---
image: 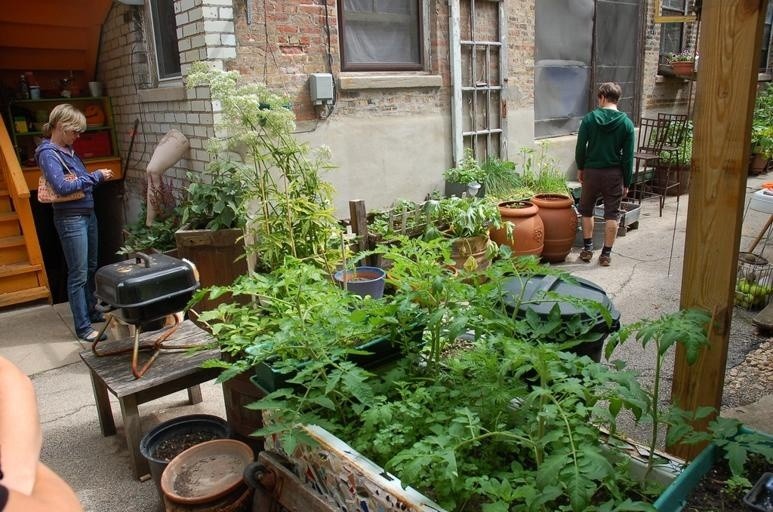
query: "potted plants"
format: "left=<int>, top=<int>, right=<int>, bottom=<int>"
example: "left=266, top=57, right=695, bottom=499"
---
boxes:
left=651, top=401, right=773, bottom=512
left=241, top=242, right=692, bottom=512
left=651, top=79, right=773, bottom=195
left=175, top=158, right=251, bottom=325
left=115, top=171, right=178, bottom=259
left=742, top=472, right=773, bottom=512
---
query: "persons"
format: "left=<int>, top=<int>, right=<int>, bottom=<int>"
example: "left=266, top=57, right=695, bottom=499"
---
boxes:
left=575, top=81, right=635, bottom=266
left=34, top=104, right=113, bottom=341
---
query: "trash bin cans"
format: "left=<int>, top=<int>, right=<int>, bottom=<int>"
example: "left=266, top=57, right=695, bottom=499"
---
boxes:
left=492, top=270, right=620, bottom=424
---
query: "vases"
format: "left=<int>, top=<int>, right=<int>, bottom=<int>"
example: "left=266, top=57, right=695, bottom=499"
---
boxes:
left=87, top=81, right=103, bottom=96
left=671, top=62, right=694, bottom=76
left=138, top=413, right=255, bottom=512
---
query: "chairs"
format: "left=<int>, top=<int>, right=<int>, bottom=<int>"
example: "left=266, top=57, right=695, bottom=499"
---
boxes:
left=623, top=117, right=671, bottom=217
left=639, top=113, right=688, bottom=208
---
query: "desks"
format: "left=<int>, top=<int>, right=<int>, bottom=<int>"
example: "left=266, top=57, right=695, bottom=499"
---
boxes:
left=79, top=323, right=222, bottom=480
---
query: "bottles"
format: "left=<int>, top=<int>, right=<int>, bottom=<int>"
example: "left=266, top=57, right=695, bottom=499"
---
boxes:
left=18, top=74, right=30, bottom=100
left=23, top=71, right=41, bottom=100
left=57, top=78, right=72, bottom=98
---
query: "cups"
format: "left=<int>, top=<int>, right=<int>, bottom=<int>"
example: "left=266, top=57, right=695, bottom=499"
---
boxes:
left=86, top=82, right=104, bottom=98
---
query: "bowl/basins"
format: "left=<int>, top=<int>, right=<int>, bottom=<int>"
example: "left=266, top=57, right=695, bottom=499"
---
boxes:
left=31, top=122, right=47, bottom=131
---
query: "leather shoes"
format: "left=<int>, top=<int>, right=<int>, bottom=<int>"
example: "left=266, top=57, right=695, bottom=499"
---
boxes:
left=78, top=316, right=108, bottom=342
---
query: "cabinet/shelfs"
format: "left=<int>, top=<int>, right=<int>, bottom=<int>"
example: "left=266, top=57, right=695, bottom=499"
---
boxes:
left=5, top=96, right=123, bottom=193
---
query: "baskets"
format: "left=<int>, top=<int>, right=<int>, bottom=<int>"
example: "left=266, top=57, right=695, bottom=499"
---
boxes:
left=732, top=255, right=773, bottom=312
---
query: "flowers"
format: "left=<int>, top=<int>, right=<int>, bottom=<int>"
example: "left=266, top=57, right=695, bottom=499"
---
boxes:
left=663, top=48, right=694, bottom=61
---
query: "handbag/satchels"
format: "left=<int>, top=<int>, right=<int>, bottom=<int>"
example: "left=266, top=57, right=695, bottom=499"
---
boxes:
left=36, top=174, right=85, bottom=204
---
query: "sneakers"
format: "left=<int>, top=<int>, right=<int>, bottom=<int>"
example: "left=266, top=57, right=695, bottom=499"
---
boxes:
left=598, top=254, right=611, bottom=266
left=580, top=249, right=592, bottom=261
